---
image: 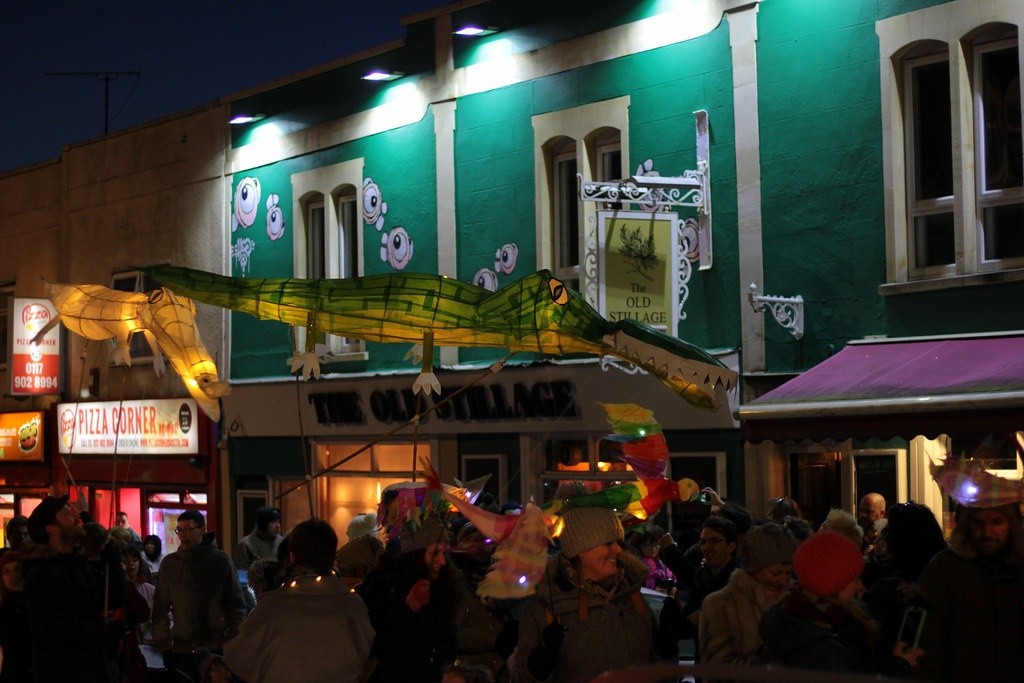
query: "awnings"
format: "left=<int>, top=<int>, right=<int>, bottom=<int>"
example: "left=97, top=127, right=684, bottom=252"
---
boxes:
left=733, top=329, right=1024, bottom=421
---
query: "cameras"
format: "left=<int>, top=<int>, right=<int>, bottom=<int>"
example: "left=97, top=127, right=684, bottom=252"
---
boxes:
left=700, top=489, right=706, bottom=503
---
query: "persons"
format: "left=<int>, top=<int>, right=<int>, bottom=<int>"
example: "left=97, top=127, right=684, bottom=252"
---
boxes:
left=0, top=472, right=1024, bottom=683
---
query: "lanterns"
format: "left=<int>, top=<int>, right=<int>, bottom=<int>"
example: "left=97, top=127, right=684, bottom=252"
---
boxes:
left=30, top=262, right=738, bottom=422
left=371, top=403, right=699, bottom=604
left=929, top=431, right=1024, bottom=509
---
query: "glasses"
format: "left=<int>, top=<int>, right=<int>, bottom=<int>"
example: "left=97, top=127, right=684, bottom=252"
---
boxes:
left=697, top=537, right=725, bottom=545
left=175, top=527, right=197, bottom=534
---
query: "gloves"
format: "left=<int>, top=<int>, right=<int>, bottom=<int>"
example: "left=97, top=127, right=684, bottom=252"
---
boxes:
left=542, top=619, right=570, bottom=655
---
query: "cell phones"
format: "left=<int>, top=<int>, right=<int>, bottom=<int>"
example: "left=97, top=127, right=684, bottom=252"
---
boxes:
left=896, top=605, right=926, bottom=653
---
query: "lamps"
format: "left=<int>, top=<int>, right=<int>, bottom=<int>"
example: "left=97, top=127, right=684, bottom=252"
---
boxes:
left=228, top=113, right=265, bottom=126
left=450, top=22, right=499, bottom=37
left=360, top=68, right=403, bottom=82
left=286, top=313, right=320, bottom=382
left=413, top=330, right=441, bottom=397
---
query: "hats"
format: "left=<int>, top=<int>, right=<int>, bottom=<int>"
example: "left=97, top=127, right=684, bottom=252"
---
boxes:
left=192, top=646, right=224, bottom=683
left=817, top=507, right=863, bottom=547
left=450, top=516, right=468, bottom=536
left=477, top=492, right=501, bottom=515
left=401, top=505, right=448, bottom=552
left=744, top=523, right=798, bottom=576
left=248, top=555, right=286, bottom=590
left=247, top=507, right=281, bottom=523
left=27, top=495, right=69, bottom=544
left=552, top=480, right=625, bottom=559
left=0, top=551, right=27, bottom=575
left=345, top=513, right=376, bottom=537
left=792, top=531, right=864, bottom=597
left=340, top=534, right=373, bottom=566
